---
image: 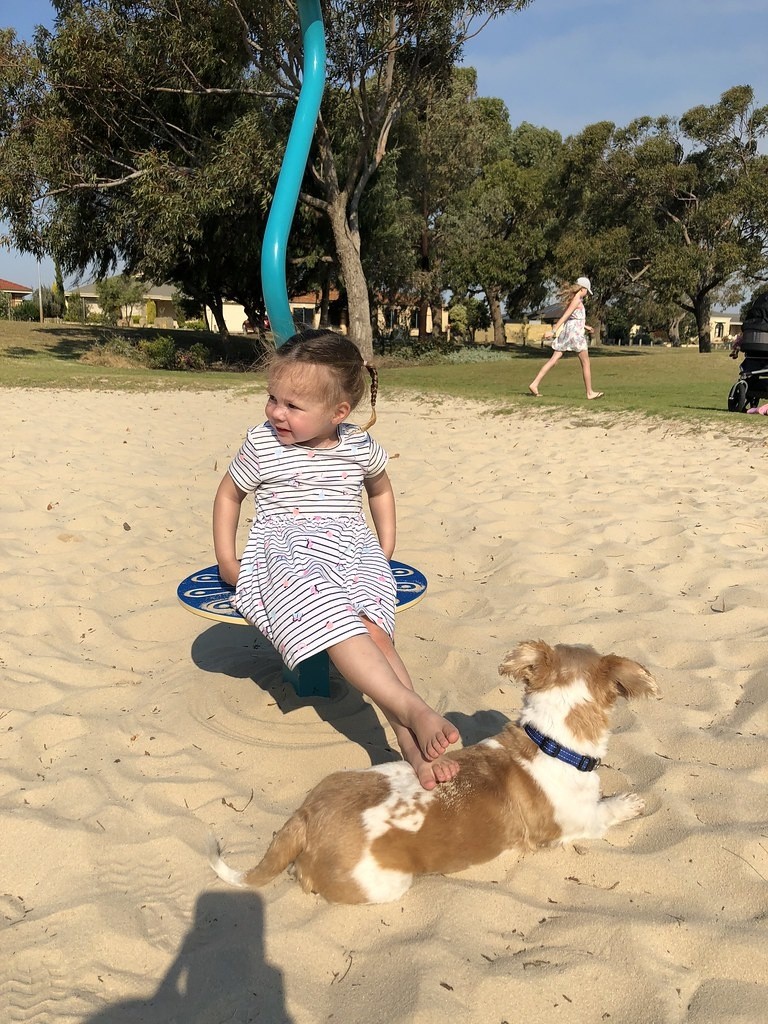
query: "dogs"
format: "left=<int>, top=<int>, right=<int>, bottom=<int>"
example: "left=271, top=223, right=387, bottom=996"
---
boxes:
left=206, top=639, right=659, bottom=907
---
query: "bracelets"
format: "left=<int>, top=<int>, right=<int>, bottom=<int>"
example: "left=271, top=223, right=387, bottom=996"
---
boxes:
left=551, top=330, right=556, bottom=333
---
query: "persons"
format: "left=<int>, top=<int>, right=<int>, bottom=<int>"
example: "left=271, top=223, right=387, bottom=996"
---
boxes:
left=528, top=276, right=605, bottom=400
left=211, top=327, right=461, bottom=792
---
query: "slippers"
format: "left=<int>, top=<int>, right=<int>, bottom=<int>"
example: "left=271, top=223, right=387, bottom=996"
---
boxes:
left=528, top=393, right=540, bottom=397
left=589, top=392, right=604, bottom=400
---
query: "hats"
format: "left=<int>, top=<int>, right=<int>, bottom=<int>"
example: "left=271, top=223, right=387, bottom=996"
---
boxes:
left=575, top=277, right=593, bottom=295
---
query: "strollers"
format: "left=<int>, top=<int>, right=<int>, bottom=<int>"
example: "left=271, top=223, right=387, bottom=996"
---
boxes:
left=727, top=290, right=768, bottom=412
